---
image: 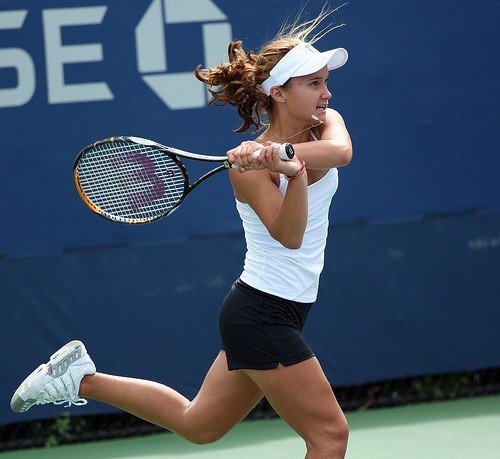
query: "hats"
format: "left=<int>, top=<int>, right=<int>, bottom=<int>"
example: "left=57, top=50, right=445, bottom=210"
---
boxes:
left=260, top=43, right=349, bottom=96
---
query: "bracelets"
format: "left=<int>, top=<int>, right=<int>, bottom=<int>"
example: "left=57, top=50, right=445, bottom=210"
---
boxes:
left=284, top=159, right=306, bottom=181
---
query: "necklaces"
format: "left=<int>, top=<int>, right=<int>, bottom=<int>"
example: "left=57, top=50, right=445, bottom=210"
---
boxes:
left=270, top=114, right=323, bottom=139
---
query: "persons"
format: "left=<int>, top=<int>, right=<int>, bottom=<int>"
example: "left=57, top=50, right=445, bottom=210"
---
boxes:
left=10, top=37, right=354, bottom=459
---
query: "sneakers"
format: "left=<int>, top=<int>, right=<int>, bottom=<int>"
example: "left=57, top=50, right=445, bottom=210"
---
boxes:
left=10, top=341, right=96, bottom=414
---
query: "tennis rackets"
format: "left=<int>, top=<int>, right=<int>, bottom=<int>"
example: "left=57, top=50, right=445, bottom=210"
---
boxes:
left=70, top=134, right=296, bottom=227
